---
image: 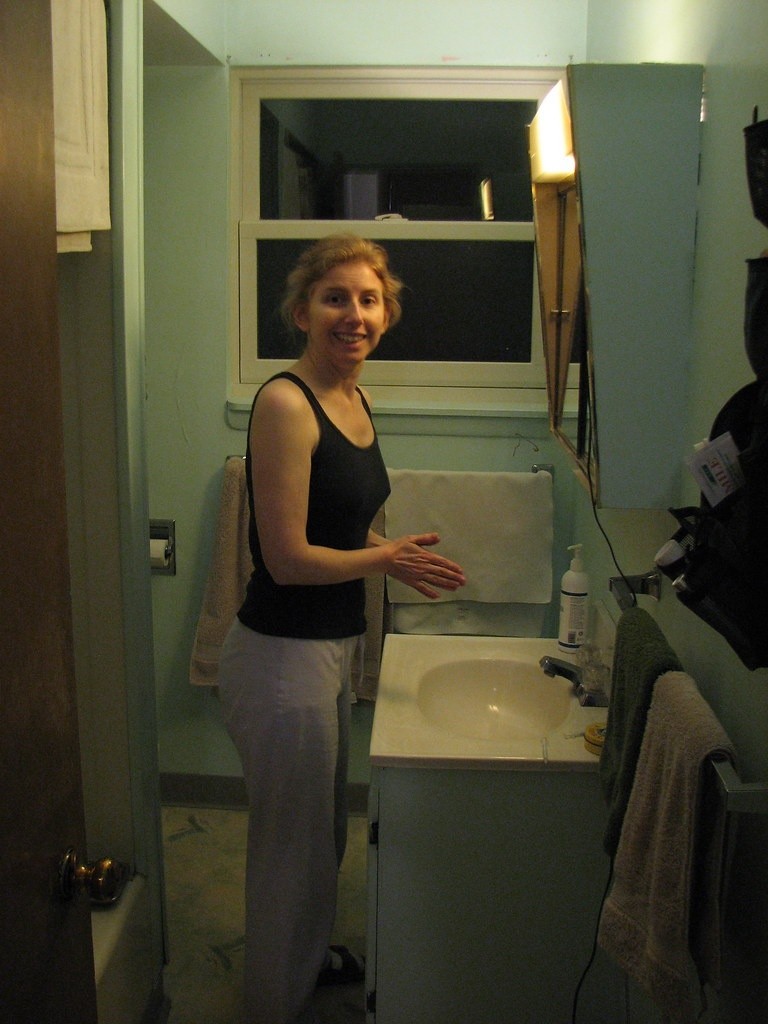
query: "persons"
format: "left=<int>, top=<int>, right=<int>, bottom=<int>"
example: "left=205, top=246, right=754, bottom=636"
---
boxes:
left=216, top=238, right=468, bottom=1024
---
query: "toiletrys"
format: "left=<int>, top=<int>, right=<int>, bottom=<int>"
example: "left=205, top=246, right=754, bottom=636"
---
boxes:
left=557, top=542, right=592, bottom=653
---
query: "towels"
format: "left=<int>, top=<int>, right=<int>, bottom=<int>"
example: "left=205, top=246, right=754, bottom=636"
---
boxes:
left=190, top=455, right=557, bottom=705
left=575, top=606, right=743, bottom=1024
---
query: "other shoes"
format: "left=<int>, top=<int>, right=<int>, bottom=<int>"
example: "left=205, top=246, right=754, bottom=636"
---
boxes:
left=316, top=944, right=364, bottom=986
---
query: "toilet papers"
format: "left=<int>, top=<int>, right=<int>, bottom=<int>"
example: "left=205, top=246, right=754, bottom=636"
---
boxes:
left=150, top=536, right=170, bottom=565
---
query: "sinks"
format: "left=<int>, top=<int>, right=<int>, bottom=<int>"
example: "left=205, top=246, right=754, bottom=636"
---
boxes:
left=416, top=659, right=573, bottom=742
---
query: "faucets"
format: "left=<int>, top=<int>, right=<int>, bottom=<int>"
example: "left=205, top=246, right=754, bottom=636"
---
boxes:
left=539, top=655, right=586, bottom=686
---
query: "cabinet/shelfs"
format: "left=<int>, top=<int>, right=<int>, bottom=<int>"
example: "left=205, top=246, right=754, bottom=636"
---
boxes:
left=0, top=0, right=169, bottom=1024
left=524, top=62, right=706, bottom=513
